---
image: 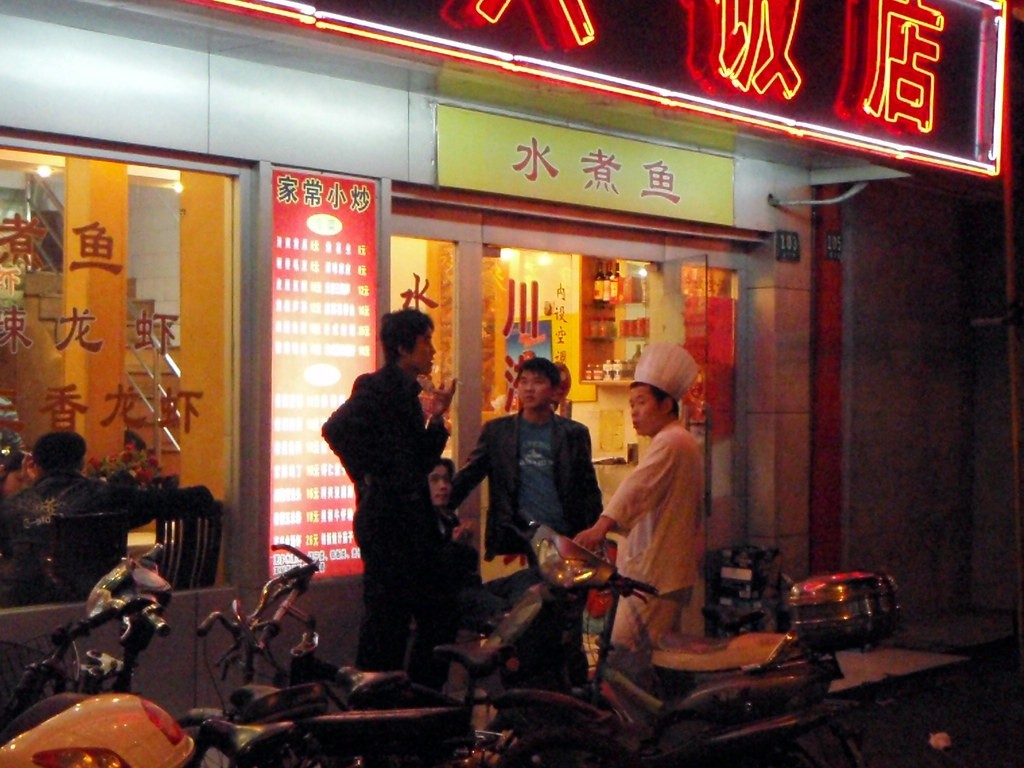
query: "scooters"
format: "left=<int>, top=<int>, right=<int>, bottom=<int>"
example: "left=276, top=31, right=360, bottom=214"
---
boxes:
left=430, top=517, right=903, bottom=767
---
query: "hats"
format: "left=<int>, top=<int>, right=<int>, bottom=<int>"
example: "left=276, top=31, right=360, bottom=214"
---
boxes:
left=632, top=343, right=698, bottom=402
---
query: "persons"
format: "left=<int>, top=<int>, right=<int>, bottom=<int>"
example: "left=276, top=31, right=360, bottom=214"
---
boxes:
left=321, top=309, right=480, bottom=691
left=444, top=357, right=604, bottom=569
left=573, top=341, right=707, bottom=646
left=0, top=429, right=153, bottom=610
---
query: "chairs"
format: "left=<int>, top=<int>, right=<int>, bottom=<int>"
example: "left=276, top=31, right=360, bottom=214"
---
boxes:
left=152, top=486, right=225, bottom=589
left=50, top=510, right=130, bottom=604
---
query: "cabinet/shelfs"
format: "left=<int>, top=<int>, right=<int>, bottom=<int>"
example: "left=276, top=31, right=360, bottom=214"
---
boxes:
left=576, top=255, right=652, bottom=387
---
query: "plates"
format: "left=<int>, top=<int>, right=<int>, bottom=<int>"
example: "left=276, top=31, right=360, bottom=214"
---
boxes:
left=307, top=214, right=342, bottom=235
left=303, top=363, right=341, bottom=388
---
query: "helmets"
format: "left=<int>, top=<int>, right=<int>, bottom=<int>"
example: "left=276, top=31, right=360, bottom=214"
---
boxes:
left=0, top=428, right=24, bottom=480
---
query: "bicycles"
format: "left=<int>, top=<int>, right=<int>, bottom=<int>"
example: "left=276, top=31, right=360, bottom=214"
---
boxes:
left=0, top=537, right=799, bottom=768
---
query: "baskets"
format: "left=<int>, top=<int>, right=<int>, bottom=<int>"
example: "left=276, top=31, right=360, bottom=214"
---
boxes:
left=0, top=640, right=51, bottom=722
